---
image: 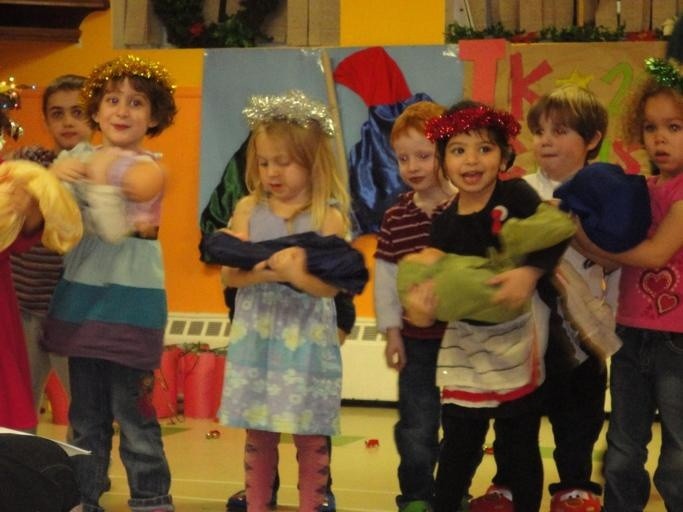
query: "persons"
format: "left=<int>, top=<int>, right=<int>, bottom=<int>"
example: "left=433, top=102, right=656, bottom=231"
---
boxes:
left=547, top=53, right=683, bottom=511
left=514, top=75, right=623, bottom=512
left=393, top=98, right=573, bottom=510
left=0, top=76, right=47, bottom=435
left=5, top=73, right=93, bottom=428
left=372, top=96, right=478, bottom=512
left=39, top=52, right=177, bottom=510
left=197, top=85, right=370, bottom=512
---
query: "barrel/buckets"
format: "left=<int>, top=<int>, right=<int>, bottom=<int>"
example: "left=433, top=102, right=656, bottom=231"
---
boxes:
left=183, top=349, right=227, bottom=422
left=146, top=345, right=183, bottom=421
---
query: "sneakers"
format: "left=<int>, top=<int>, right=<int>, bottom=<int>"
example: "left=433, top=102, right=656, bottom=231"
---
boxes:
left=470, top=483, right=515, bottom=512
left=549, top=482, right=599, bottom=511
left=318, top=492, right=335, bottom=510
left=226, top=489, right=277, bottom=509
